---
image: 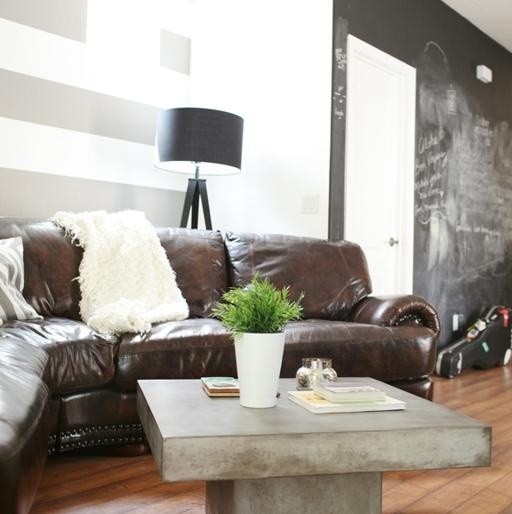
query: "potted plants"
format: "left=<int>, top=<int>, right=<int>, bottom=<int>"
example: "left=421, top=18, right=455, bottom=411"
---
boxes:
left=208, top=266, right=306, bottom=407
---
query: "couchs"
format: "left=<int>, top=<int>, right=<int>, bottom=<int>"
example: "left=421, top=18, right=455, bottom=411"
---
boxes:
left=0, top=220, right=442, bottom=513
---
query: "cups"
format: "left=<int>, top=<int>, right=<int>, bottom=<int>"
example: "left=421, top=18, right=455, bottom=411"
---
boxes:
left=301, top=356, right=332, bottom=385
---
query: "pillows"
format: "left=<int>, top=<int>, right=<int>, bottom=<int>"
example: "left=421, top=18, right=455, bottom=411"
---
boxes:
left=0, top=235, right=45, bottom=320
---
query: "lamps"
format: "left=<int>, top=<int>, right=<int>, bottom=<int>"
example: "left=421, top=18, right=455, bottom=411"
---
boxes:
left=152, top=105, right=246, bottom=229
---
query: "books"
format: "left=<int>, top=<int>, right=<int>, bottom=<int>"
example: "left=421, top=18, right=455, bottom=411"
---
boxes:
left=314, top=386, right=386, bottom=401
left=290, top=390, right=407, bottom=415
left=200, top=376, right=239, bottom=397
